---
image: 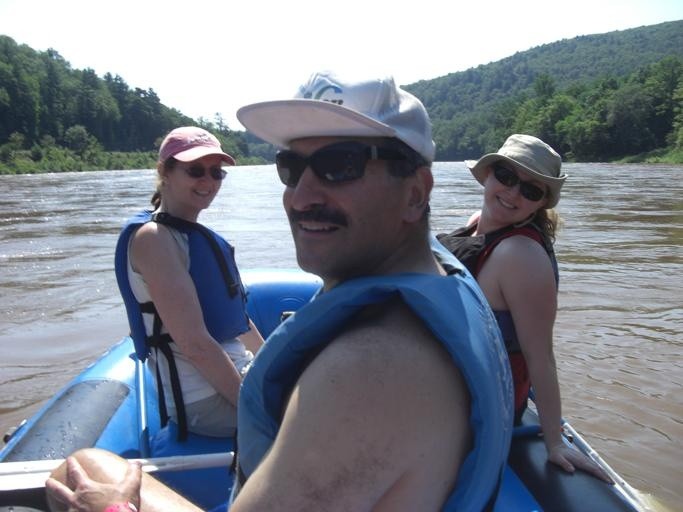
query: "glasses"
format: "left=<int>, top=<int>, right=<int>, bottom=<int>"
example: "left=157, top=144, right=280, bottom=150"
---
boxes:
left=275, top=140, right=408, bottom=189
left=185, top=165, right=228, bottom=180
left=493, top=164, right=544, bottom=201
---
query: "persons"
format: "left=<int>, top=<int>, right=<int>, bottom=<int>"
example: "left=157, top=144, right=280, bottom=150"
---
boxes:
left=45, top=70, right=516, bottom=512
left=114, top=127, right=265, bottom=440
left=433, top=134, right=617, bottom=484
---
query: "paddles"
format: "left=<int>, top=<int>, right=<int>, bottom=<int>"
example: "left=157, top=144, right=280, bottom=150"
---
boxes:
left=1, top=452, right=233, bottom=491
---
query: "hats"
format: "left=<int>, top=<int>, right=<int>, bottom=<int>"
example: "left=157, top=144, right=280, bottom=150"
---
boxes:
left=236, top=60, right=437, bottom=162
left=464, top=134, right=568, bottom=209
left=159, top=125, right=236, bottom=165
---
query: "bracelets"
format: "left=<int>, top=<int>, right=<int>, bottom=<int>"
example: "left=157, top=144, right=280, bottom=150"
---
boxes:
left=105, top=501, right=138, bottom=512
